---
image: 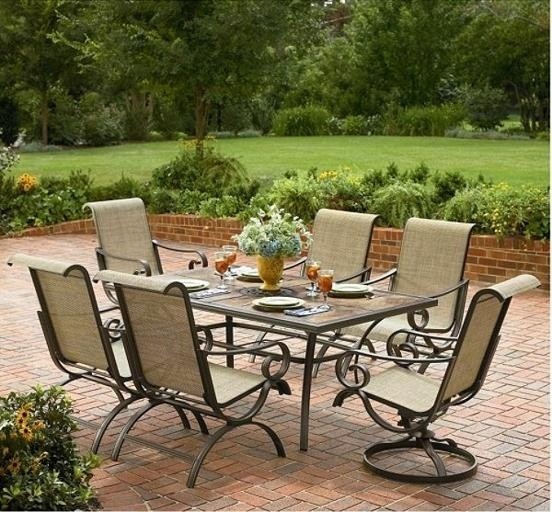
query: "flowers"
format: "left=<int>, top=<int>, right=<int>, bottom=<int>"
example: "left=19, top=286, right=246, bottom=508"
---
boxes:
left=232, top=202, right=312, bottom=256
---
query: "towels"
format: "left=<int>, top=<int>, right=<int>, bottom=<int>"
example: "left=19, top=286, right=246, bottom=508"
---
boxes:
left=283, top=303, right=331, bottom=318
left=188, top=282, right=230, bottom=298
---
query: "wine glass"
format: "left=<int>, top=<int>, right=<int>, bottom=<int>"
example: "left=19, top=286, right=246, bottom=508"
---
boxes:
left=214, top=252, right=229, bottom=289
left=304, top=261, right=321, bottom=297
left=223, top=245, right=237, bottom=281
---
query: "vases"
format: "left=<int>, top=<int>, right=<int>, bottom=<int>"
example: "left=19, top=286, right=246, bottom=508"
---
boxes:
left=255, top=250, right=287, bottom=293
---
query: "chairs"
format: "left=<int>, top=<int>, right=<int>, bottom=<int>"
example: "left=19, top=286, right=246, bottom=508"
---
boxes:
left=334, top=215, right=482, bottom=388
left=6, top=251, right=215, bottom=460
left=330, top=271, right=544, bottom=489
left=246, top=207, right=379, bottom=380
left=92, top=266, right=295, bottom=492
left=79, top=194, right=210, bottom=343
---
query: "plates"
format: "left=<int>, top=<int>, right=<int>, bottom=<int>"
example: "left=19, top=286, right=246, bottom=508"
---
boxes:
left=170, top=268, right=373, bottom=309
